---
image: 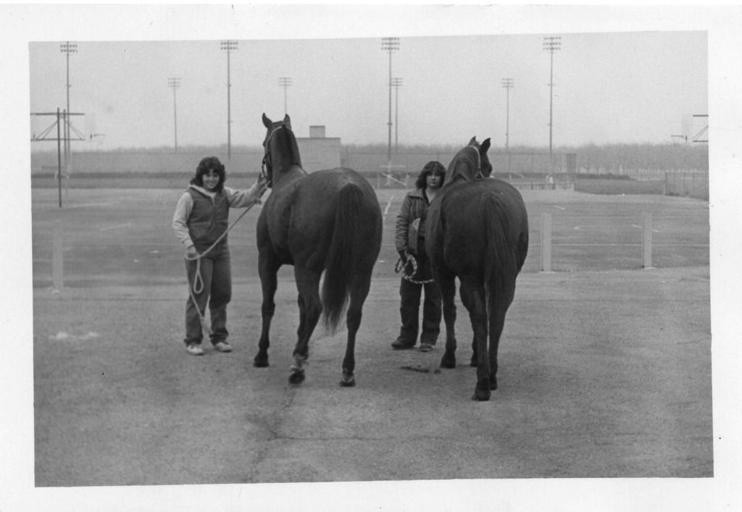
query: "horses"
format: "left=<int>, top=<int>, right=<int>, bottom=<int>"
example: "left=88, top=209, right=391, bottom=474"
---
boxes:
left=421, top=133, right=531, bottom=403
left=251, top=111, right=385, bottom=387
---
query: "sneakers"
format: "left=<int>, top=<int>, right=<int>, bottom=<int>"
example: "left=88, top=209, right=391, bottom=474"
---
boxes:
left=214, top=342, right=233, bottom=351
left=391, top=337, right=415, bottom=350
left=420, top=342, right=435, bottom=352
left=186, top=343, right=204, bottom=356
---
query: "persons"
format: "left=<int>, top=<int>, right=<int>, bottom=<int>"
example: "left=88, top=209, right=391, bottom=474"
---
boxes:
left=390, top=161, right=448, bottom=352
left=172, top=156, right=271, bottom=356
